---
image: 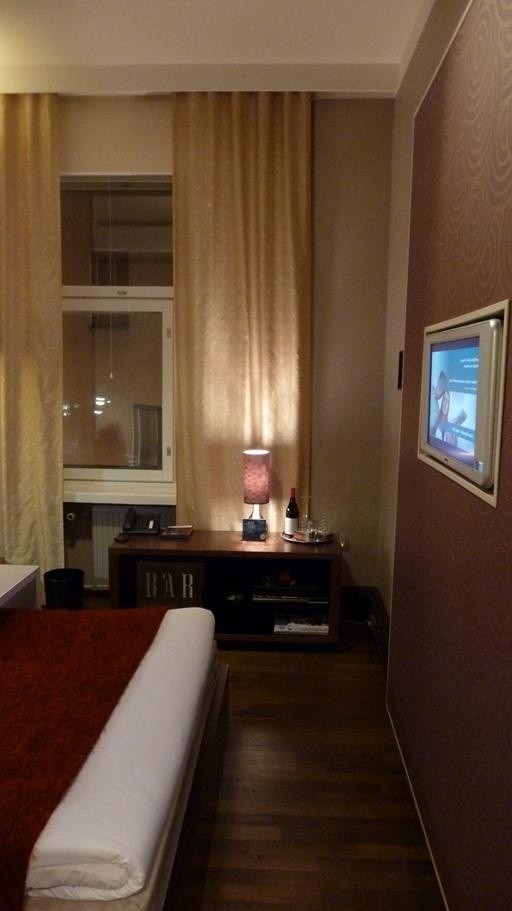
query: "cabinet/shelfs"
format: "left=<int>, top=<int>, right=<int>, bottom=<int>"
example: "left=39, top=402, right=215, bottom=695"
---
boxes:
left=106, top=528, right=344, bottom=653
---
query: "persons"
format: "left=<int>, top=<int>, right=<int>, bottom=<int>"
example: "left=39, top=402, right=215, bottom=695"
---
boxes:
left=429, top=370, right=467, bottom=445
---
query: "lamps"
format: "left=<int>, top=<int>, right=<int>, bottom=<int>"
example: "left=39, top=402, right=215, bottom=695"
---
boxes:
left=239, top=449, right=271, bottom=542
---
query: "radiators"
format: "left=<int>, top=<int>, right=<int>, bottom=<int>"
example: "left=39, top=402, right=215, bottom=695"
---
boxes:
left=91, top=506, right=174, bottom=579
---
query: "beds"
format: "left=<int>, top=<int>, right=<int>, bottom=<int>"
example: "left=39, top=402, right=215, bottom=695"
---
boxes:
left=1, top=606, right=230, bottom=911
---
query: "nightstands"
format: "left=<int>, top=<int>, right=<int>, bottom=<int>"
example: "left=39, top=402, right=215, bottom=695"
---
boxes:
left=0, top=563, right=42, bottom=608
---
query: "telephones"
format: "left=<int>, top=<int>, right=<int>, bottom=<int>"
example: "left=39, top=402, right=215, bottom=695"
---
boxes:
left=123, top=508, right=161, bottom=534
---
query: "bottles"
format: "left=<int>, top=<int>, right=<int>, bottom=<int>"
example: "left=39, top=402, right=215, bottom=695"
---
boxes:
left=284, top=487, right=299, bottom=539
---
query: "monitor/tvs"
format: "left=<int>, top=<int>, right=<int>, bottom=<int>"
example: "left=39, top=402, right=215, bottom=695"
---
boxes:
left=420, top=317, right=503, bottom=491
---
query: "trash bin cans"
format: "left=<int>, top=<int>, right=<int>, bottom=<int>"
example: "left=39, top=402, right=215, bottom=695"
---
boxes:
left=44, top=568, right=85, bottom=611
